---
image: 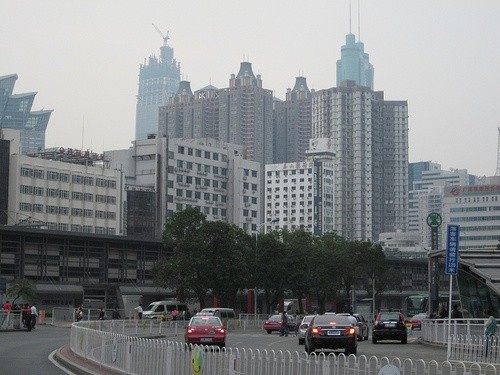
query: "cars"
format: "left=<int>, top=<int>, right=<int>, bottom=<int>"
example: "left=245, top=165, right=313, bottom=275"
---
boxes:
left=293, top=311, right=370, bottom=356
left=263, top=314, right=301, bottom=335
left=409, top=300, right=479, bottom=331
left=183, top=315, right=227, bottom=352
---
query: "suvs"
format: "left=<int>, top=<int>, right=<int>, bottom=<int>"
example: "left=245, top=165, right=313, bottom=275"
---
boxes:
left=371, top=312, right=409, bottom=344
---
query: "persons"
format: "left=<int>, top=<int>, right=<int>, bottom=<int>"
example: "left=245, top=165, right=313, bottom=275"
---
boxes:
left=185, top=309, right=191, bottom=320
left=280, top=311, right=288, bottom=337
left=13, top=304, right=38, bottom=331
left=348, top=311, right=357, bottom=325
left=138, top=304, right=142, bottom=319
left=172, top=308, right=177, bottom=320
left=451, top=305, right=462, bottom=323
left=294, top=312, right=301, bottom=337
left=99, top=308, right=105, bottom=320
left=481, top=310, right=496, bottom=356
left=1, top=301, right=12, bottom=325
left=76, top=307, right=83, bottom=321
left=439, top=304, right=448, bottom=323
left=112, top=307, right=119, bottom=319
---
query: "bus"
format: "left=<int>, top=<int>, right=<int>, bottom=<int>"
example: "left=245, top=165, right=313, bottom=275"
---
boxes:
left=405, top=295, right=450, bottom=318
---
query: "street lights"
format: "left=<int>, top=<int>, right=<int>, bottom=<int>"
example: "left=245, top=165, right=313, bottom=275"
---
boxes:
left=372, top=237, right=393, bottom=321
left=254, top=218, right=279, bottom=314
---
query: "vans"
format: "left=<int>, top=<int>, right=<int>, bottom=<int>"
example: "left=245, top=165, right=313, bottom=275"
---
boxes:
left=140, top=300, right=191, bottom=324
left=199, top=307, right=237, bottom=330
